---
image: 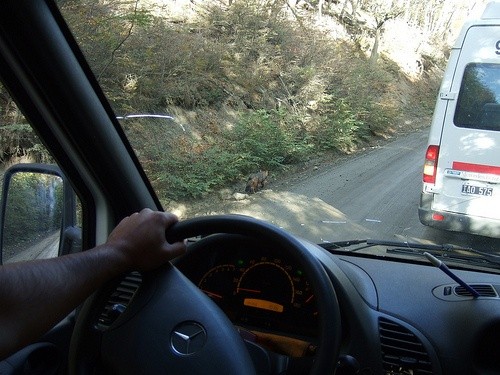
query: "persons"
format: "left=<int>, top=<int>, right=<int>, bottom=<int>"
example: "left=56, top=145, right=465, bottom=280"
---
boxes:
left=0, top=208, right=190, bottom=374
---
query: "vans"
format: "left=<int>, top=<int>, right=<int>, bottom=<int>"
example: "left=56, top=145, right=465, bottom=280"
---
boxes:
left=417, top=16, right=500, bottom=240
